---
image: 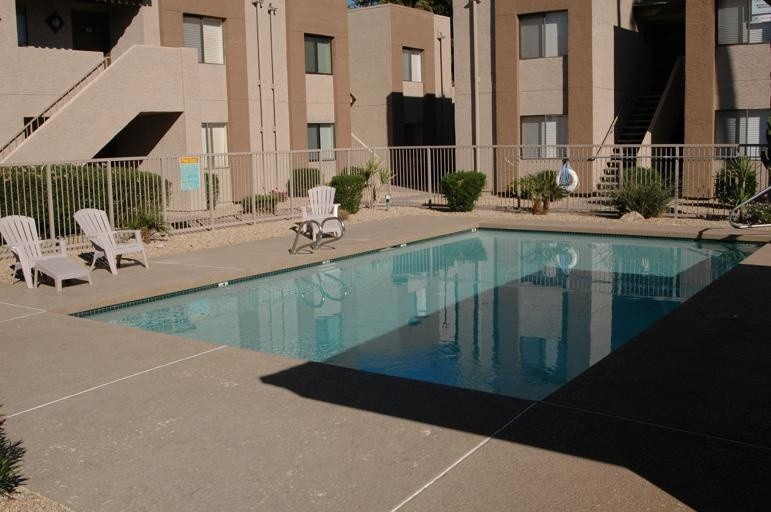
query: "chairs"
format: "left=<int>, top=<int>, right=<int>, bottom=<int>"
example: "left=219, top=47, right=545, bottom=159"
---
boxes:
left=0, top=208, right=148, bottom=288
left=298, top=185, right=341, bottom=233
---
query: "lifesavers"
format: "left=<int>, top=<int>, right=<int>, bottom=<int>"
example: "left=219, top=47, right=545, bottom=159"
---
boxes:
left=556, top=169, right=577, bottom=192
left=555, top=248, right=577, bottom=271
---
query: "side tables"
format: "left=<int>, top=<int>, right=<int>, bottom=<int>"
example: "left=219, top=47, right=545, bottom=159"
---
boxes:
left=33, top=255, right=93, bottom=293
left=310, top=216, right=340, bottom=242
